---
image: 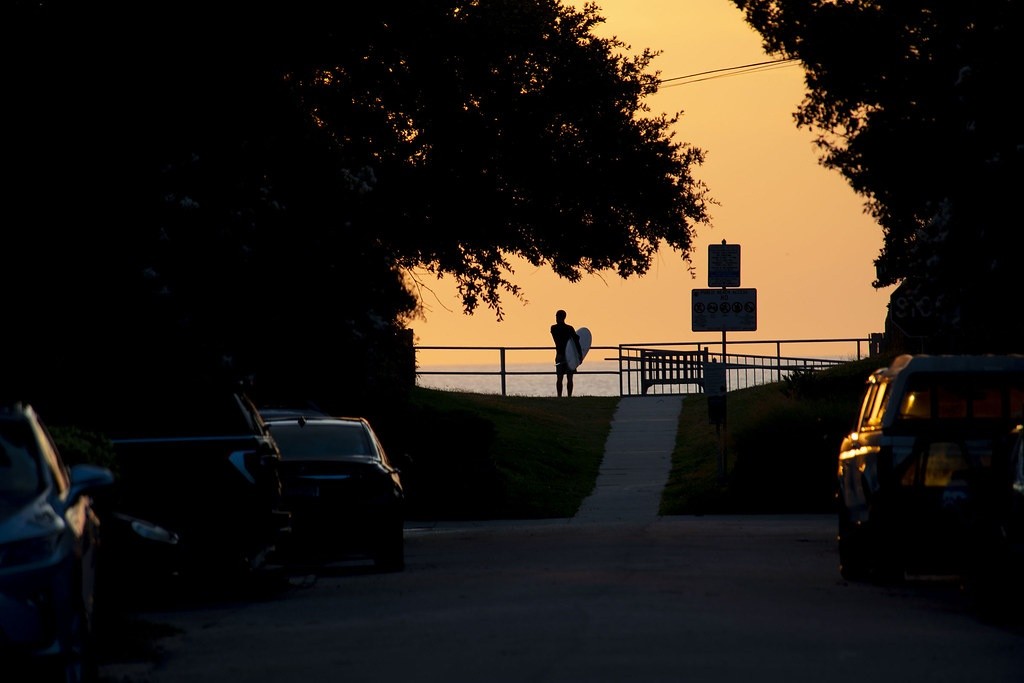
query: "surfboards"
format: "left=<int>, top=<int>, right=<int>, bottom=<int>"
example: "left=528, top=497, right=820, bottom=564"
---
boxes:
left=564, top=327, right=592, bottom=373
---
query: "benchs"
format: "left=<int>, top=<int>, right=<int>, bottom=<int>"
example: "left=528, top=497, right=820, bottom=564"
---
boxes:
left=641, top=347, right=708, bottom=393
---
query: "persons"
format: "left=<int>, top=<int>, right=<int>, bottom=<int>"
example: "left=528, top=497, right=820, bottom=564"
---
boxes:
left=551, top=310, right=583, bottom=397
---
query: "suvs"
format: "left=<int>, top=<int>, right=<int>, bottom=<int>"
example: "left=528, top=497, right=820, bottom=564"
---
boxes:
left=108, top=372, right=410, bottom=586
left=836, top=352, right=1024, bottom=580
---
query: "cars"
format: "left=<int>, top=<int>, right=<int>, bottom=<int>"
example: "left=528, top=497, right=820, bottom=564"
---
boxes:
left=0, top=400, right=120, bottom=683
